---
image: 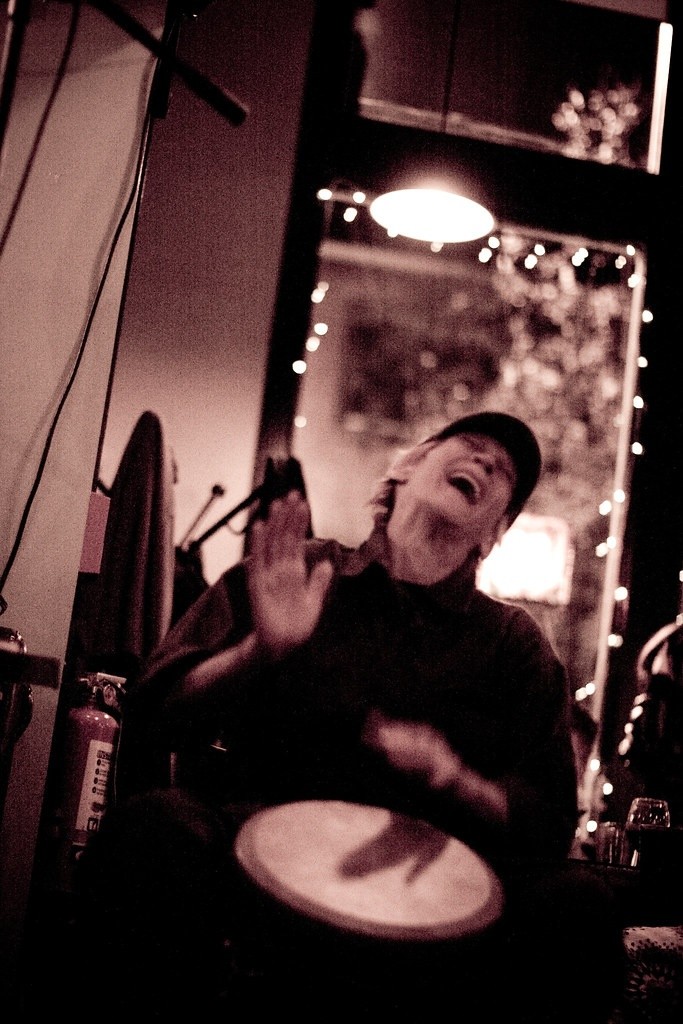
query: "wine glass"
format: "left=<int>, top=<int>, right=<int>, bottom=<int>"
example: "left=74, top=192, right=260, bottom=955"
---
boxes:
left=625, top=797, right=670, bottom=867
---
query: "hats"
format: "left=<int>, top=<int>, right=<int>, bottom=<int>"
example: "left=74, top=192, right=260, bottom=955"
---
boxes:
left=419, top=411, right=543, bottom=532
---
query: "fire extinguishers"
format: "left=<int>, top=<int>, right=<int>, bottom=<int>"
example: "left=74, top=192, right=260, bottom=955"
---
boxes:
left=61, top=673, right=128, bottom=860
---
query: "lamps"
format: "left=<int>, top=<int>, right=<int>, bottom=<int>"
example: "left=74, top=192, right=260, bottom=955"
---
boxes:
left=362, top=0, right=499, bottom=245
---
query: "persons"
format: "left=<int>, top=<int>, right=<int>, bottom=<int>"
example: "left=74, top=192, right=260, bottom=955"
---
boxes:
left=62, top=410, right=580, bottom=1024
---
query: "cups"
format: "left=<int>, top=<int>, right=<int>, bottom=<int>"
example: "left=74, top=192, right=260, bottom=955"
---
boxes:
left=596, top=823, right=624, bottom=865
